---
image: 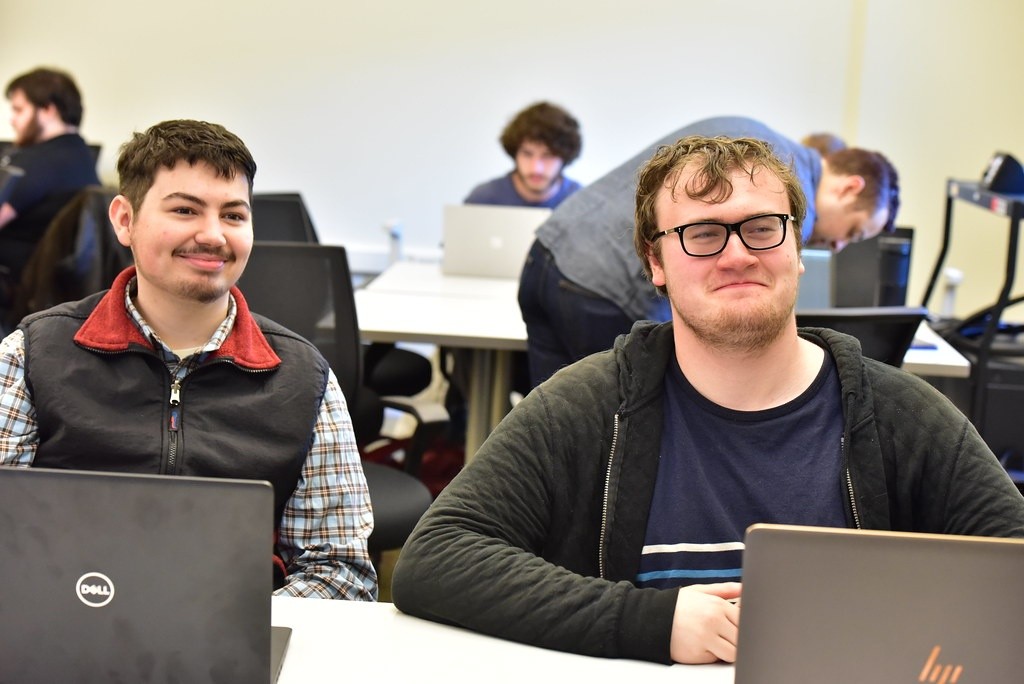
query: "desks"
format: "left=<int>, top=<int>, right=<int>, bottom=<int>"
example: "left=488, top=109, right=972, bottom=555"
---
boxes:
left=270, top=593, right=736, bottom=684
left=316, top=261, right=972, bottom=466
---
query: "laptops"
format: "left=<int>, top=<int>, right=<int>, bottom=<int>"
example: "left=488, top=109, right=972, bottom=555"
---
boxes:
left=441, top=204, right=552, bottom=280
left=0, top=465, right=291, bottom=684
left=733, top=523, right=1024, bottom=684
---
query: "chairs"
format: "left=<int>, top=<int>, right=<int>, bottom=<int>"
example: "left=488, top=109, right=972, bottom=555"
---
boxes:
left=237, top=191, right=433, bottom=572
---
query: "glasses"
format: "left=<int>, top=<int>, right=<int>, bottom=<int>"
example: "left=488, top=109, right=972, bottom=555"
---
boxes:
left=651, top=213, right=794, bottom=257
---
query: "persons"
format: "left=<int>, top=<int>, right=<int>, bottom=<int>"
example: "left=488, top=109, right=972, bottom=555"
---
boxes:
left=0, top=67, right=105, bottom=329
left=391, top=135, right=1024, bottom=666
left=464, top=102, right=900, bottom=397
left=0, top=120, right=380, bottom=603
